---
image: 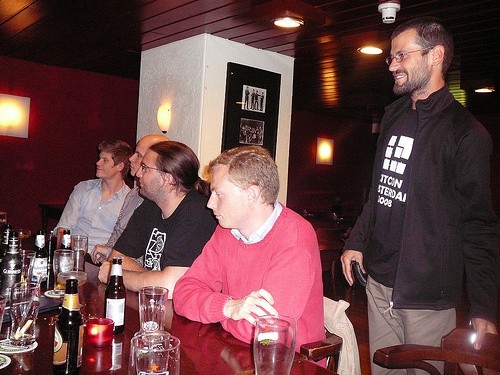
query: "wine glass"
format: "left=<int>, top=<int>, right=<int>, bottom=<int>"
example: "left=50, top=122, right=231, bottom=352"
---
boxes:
left=16, top=226, right=31, bottom=250
left=56, top=271, right=88, bottom=308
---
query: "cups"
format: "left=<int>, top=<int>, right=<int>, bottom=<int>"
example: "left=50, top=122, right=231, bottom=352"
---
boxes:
left=9, top=281, right=40, bottom=345
left=0, top=296, right=7, bottom=334
left=138, top=285, right=169, bottom=332
left=53, top=249, right=74, bottom=295
left=86, top=318, right=115, bottom=348
left=128, top=333, right=181, bottom=375
left=252, top=314, right=296, bottom=375
left=71, top=234, right=88, bottom=272
left=82, top=346, right=113, bottom=375
left=19, top=250, right=36, bottom=283
left=134, top=330, right=170, bottom=375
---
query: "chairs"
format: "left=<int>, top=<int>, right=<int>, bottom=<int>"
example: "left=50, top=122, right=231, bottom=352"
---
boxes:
left=301, top=297, right=341, bottom=373
left=373, top=325, right=500, bottom=375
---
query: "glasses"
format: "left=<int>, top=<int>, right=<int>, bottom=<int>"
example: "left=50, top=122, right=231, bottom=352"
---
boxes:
left=386, top=47, right=433, bottom=66
left=140, top=164, right=168, bottom=174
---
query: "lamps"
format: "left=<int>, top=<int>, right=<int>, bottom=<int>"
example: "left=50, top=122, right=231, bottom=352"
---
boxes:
left=157, top=106, right=171, bottom=134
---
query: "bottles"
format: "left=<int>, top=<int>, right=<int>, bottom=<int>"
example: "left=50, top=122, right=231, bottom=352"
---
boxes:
left=0, top=230, right=22, bottom=296
left=46, top=233, right=57, bottom=290
left=53, top=278, right=83, bottom=375
left=0, top=224, right=13, bottom=286
left=103, top=256, right=126, bottom=335
left=57, top=227, right=73, bottom=250
left=31, top=228, right=49, bottom=292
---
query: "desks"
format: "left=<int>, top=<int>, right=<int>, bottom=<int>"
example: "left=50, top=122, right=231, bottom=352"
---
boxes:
left=0, top=237, right=338, bottom=375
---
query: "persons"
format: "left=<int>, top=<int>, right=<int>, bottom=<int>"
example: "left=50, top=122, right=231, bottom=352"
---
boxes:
left=85, top=135, right=172, bottom=268
left=44, top=138, right=133, bottom=269
left=98, top=141, right=222, bottom=301
left=165, top=149, right=328, bottom=375
left=335, top=18, right=500, bottom=375
